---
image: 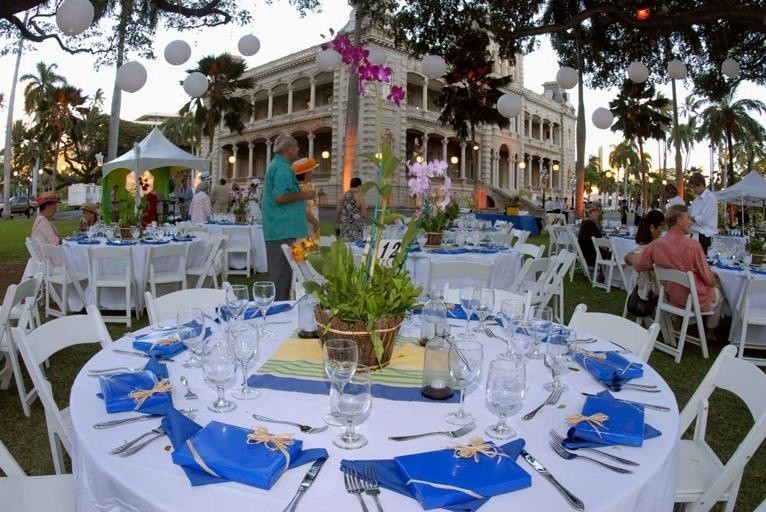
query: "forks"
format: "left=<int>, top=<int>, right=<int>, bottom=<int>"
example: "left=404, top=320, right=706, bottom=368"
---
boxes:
left=545, top=428, right=641, bottom=476
left=252, top=412, right=328, bottom=438
left=109, top=413, right=196, bottom=458
left=343, top=462, right=387, bottom=512
left=387, top=423, right=478, bottom=442
left=521, top=388, right=565, bottom=421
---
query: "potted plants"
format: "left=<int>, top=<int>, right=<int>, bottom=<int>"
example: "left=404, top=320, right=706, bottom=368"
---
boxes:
left=505, top=196, right=520, bottom=216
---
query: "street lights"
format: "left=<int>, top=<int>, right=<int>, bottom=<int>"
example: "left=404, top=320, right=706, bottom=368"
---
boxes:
left=24, top=168, right=34, bottom=219
left=540, top=165, right=551, bottom=209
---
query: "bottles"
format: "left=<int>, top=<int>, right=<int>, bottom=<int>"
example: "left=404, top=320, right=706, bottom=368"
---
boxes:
left=421, top=290, right=446, bottom=345
left=296, top=291, right=319, bottom=340
left=417, top=324, right=459, bottom=401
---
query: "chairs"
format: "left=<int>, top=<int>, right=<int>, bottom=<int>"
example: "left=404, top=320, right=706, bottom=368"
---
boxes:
left=0, top=213, right=766, bottom=512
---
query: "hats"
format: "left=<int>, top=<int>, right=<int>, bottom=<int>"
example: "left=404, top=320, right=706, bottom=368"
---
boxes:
left=34, top=192, right=62, bottom=208
left=200, top=172, right=212, bottom=180
left=585, top=204, right=598, bottom=213
left=292, top=158, right=320, bottom=176
left=79, top=203, right=100, bottom=216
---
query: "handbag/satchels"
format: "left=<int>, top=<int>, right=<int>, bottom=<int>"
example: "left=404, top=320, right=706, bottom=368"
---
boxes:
left=627, top=284, right=656, bottom=317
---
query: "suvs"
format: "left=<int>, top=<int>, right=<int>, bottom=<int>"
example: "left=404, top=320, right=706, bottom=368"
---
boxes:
left=0, top=193, right=38, bottom=217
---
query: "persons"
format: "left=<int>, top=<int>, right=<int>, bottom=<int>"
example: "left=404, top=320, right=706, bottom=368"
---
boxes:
left=663, top=183, right=686, bottom=217
left=632, top=200, right=642, bottom=226
left=289, top=156, right=324, bottom=243
left=631, top=209, right=667, bottom=300
left=336, top=176, right=368, bottom=243
left=263, top=133, right=321, bottom=300
left=688, top=173, right=719, bottom=255
left=140, top=188, right=157, bottom=228
left=578, top=205, right=612, bottom=285
left=30, top=192, right=62, bottom=271
left=108, top=184, right=122, bottom=222
left=176, top=171, right=263, bottom=226
left=734, top=207, right=751, bottom=227
left=620, top=199, right=629, bottom=225
left=624, top=204, right=728, bottom=344
left=546, top=195, right=604, bottom=224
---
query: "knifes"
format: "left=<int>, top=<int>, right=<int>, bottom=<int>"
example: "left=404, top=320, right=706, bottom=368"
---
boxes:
left=281, top=455, right=331, bottom=512
left=111, top=348, right=176, bottom=365
left=518, top=448, right=585, bottom=512
left=92, top=407, right=200, bottom=431
left=580, top=392, right=671, bottom=413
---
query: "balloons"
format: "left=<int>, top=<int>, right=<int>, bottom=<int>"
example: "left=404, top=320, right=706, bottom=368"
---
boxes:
left=77, top=202, right=103, bottom=235
left=55, top=1, right=95, bottom=39
left=164, top=40, right=191, bottom=66
left=182, top=72, right=209, bottom=99
left=365, top=44, right=387, bottom=68
left=668, top=60, right=687, bottom=80
left=628, top=62, right=649, bottom=84
left=592, top=107, right=613, bottom=129
left=721, top=58, right=740, bottom=78
left=556, top=67, right=578, bottom=89
left=237, top=35, right=261, bottom=58
left=496, top=92, right=522, bottom=120
left=314, top=48, right=341, bottom=72
left=421, top=55, right=447, bottom=81
left=116, top=61, right=147, bottom=94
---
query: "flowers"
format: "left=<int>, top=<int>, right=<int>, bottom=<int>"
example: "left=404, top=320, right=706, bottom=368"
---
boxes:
left=113, top=176, right=150, bottom=226
left=292, top=28, right=423, bottom=375
left=229, top=187, right=259, bottom=215
left=403, top=159, right=465, bottom=231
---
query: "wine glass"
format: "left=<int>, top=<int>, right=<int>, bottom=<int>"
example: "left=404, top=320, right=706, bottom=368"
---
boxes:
left=702, top=241, right=753, bottom=278
left=331, top=362, right=373, bottom=451
left=175, top=280, right=277, bottom=419
left=484, top=358, right=528, bottom=438
left=208, top=211, right=263, bottom=225
left=358, top=218, right=481, bottom=256
left=85, top=218, right=194, bottom=243
left=459, top=286, right=575, bottom=394
left=446, top=338, right=487, bottom=425
left=321, top=340, right=360, bottom=430
left=601, top=219, right=639, bottom=238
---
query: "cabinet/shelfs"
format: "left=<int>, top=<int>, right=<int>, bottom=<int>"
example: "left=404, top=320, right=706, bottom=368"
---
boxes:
left=157, top=199, right=178, bottom=226
